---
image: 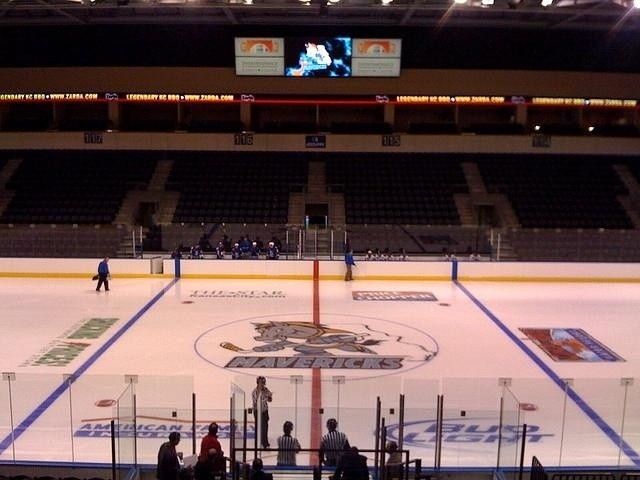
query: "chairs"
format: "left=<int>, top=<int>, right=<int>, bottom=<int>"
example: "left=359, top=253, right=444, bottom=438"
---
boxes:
left=1, top=148, right=306, bottom=226
left=321, top=150, right=640, bottom=235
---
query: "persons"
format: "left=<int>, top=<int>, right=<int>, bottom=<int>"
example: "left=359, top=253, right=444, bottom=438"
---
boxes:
left=319, top=418, right=351, bottom=466
left=156, top=431, right=181, bottom=480
left=446, top=252, right=454, bottom=261
left=201, top=421, right=221, bottom=456
left=469, top=251, right=480, bottom=261
left=328, top=444, right=368, bottom=480
left=384, top=440, right=402, bottom=479
left=96, top=256, right=111, bottom=292
left=178, top=470, right=194, bottom=480
left=248, top=458, right=273, bottom=479
left=276, top=421, right=301, bottom=467
left=252, top=376, right=273, bottom=448
left=195, top=448, right=220, bottom=479
left=365, top=248, right=408, bottom=261
left=344, top=248, right=356, bottom=281
left=171, top=235, right=282, bottom=261
left=342, top=238, right=351, bottom=253
